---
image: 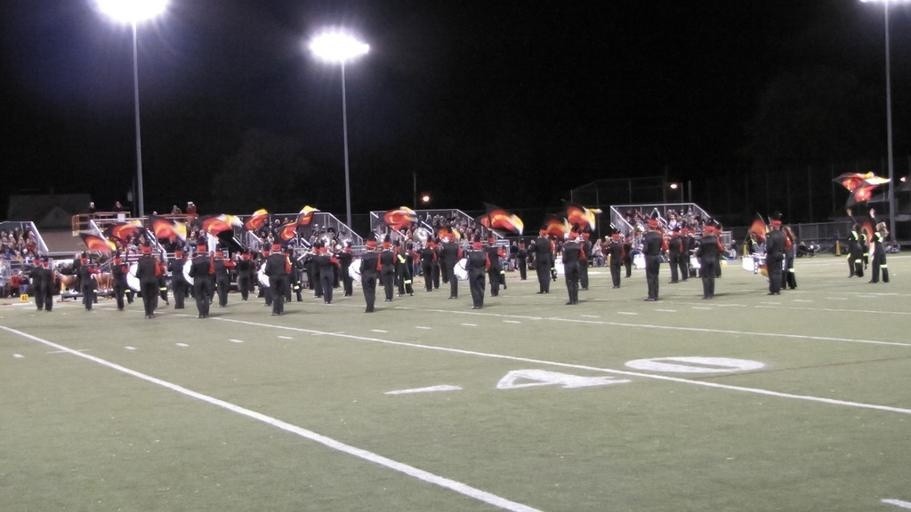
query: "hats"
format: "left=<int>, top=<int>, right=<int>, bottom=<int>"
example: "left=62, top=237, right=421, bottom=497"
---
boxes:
left=25, top=235, right=345, bottom=263
left=364, top=220, right=797, bottom=257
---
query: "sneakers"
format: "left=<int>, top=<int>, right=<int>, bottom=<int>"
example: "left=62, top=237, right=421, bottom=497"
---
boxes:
left=33, top=264, right=896, bottom=312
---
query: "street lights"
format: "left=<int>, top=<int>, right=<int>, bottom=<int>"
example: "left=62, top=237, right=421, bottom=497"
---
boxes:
left=95, top=0, right=169, bottom=218
left=859, top=0, right=911, bottom=243
left=308, top=32, right=371, bottom=230
